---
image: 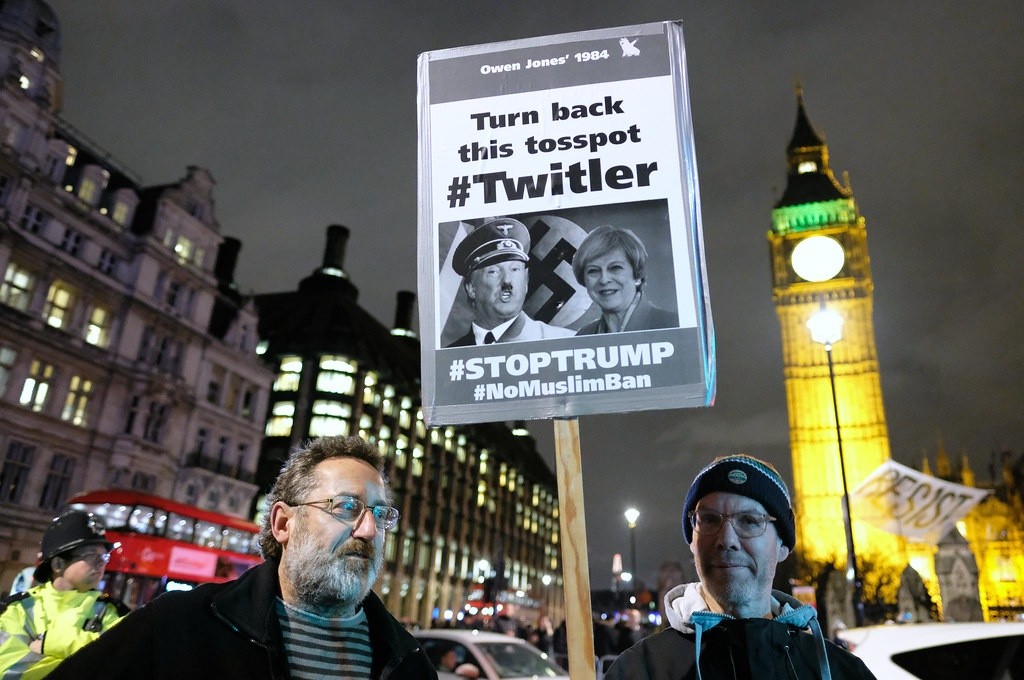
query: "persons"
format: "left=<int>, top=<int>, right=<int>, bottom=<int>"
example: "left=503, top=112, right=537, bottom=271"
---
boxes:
left=0, top=509, right=133, bottom=680
left=41, top=436, right=440, bottom=680
left=572, top=226, right=680, bottom=336
left=603, top=453, right=877, bottom=680
left=857, top=592, right=920, bottom=627
left=395, top=614, right=651, bottom=673
left=445, top=218, right=577, bottom=348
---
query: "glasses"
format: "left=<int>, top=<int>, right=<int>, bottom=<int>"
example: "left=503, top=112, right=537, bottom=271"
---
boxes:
left=290, top=495, right=401, bottom=529
left=73, top=553, right=111, bottom=567
left=686, top=508, right=777, bottom=539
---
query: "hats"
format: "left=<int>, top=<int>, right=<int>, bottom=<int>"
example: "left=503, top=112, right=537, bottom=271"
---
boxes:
left=451, top=218, right=531, bottom=279
left=682, top=454, right=796, bottom=551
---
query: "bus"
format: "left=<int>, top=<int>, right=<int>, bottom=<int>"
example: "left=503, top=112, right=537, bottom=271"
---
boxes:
left=57, top=487, right=265, bottom=617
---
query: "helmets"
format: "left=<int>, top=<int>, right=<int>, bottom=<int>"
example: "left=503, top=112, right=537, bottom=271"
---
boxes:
left=33, top=511, right=113, bottom=583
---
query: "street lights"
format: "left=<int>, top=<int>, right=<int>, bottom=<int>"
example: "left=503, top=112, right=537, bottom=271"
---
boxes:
left=624, top=501, right=640, bottom=616
left=806, top=300, right=858, bottom=585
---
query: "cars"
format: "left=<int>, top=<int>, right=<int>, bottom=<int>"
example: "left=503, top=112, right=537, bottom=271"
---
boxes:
left=838, top=622, right=1024, bottom=680
left=407, top=630, right=569, bottom=680
